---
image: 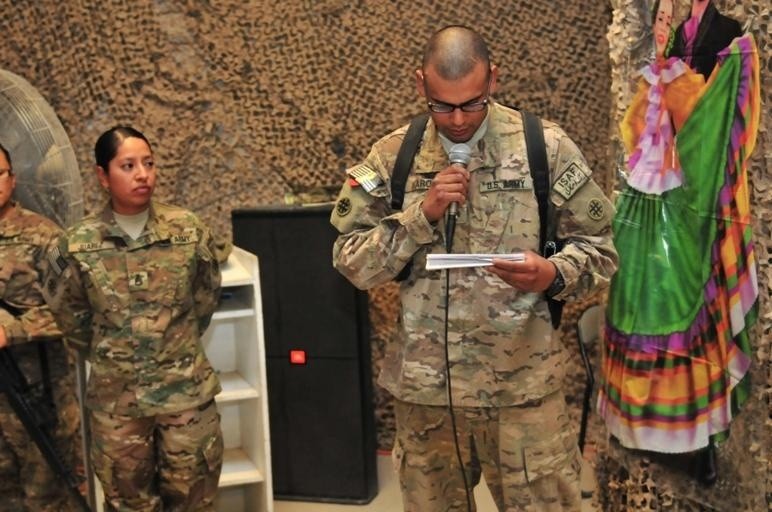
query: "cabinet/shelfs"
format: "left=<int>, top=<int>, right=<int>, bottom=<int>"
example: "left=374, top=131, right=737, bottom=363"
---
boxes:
left=85, top=244, right=274, bottom=511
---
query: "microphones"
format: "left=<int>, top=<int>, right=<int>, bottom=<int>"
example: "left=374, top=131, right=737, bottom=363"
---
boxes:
left=443, top=140, right=470, bottom=255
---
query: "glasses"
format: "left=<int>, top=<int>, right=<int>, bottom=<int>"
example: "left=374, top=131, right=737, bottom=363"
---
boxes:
left=424, top=87, right=490, bottom=113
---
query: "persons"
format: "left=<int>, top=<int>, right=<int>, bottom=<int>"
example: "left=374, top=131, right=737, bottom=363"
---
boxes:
left=58, top=126, right=226, bottom=511
left=0, top=140, right=88, bottom=511
left=328, top=20, right=621, bottom=512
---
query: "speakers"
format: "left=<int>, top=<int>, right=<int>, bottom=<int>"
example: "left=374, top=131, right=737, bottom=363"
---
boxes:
left=230, top=201, right=380, bottom=505
